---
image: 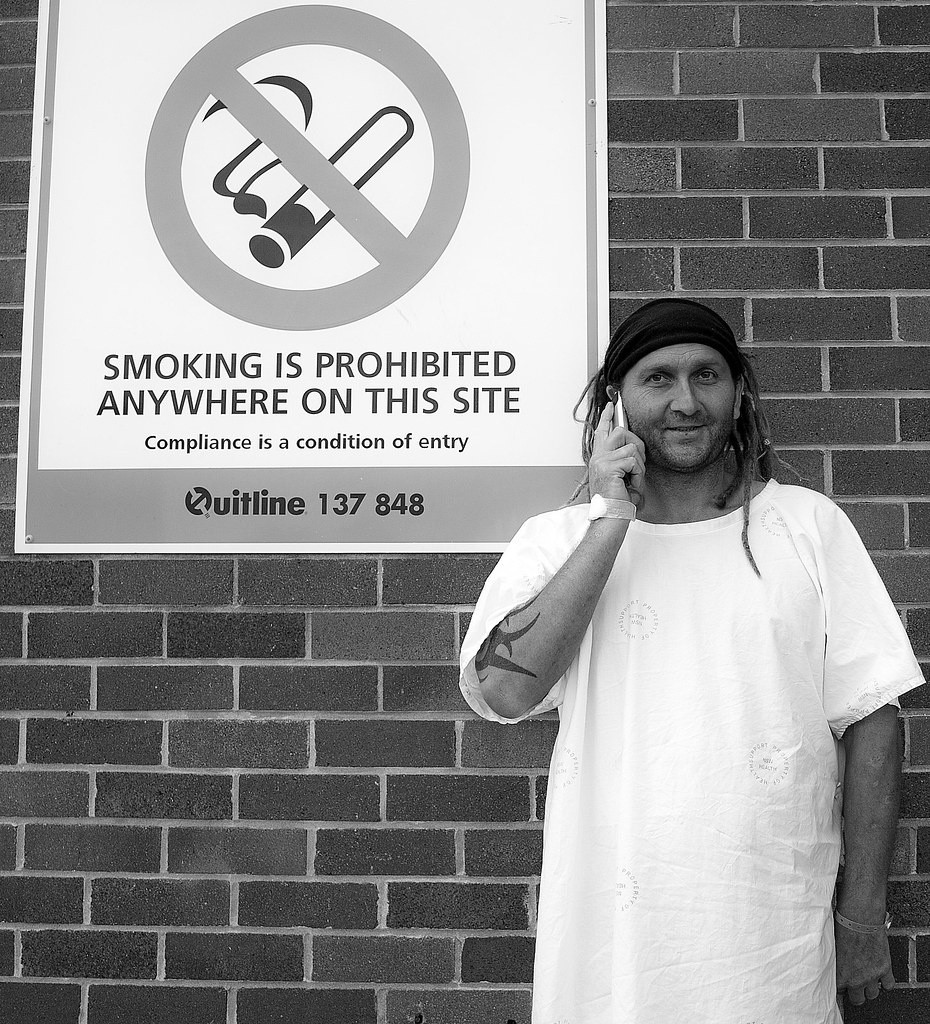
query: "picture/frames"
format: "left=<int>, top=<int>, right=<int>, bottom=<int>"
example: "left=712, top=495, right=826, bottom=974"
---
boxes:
left=12, top=0, right=613, bottom=555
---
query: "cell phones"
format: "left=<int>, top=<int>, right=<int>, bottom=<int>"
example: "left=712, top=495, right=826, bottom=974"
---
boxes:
left=608, top=390, right=628, bottom=435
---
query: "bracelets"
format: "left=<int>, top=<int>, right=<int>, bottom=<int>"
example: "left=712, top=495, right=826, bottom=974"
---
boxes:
left=835, top=908, right=894, bottom=934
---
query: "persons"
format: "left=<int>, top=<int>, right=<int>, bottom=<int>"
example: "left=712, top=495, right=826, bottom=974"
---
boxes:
left=459, top=296, right=926, bottom=1023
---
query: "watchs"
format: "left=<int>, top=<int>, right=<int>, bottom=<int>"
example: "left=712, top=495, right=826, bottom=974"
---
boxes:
left=588, top=492, right=637, bottom=523
